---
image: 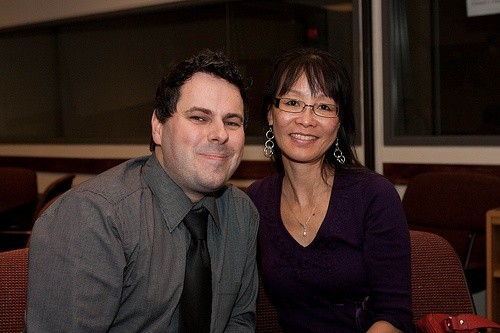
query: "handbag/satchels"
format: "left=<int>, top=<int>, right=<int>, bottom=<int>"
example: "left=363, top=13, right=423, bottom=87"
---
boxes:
left=417, top=312, right=500, bottom=333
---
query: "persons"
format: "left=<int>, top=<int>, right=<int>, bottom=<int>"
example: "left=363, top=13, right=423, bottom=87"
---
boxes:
left=25, top=48, right=261, bottom=333
left=243, top=44, right=415, bottom=333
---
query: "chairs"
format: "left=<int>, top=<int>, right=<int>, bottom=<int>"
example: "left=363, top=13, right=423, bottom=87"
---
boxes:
left=257, top=230, right=477, bottom=333
left=0, top=247, right=29, bottom=333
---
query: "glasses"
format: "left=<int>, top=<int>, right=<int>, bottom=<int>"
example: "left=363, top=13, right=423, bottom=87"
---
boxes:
left=272, top=97, right=343, bottom=118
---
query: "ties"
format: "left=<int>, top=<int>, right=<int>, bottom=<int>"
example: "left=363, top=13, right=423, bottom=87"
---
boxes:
left=168, top=224, right=214, bottom=333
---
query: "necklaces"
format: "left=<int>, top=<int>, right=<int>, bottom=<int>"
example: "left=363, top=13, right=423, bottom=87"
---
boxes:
left=280, top=169, right=327, bottom=236
